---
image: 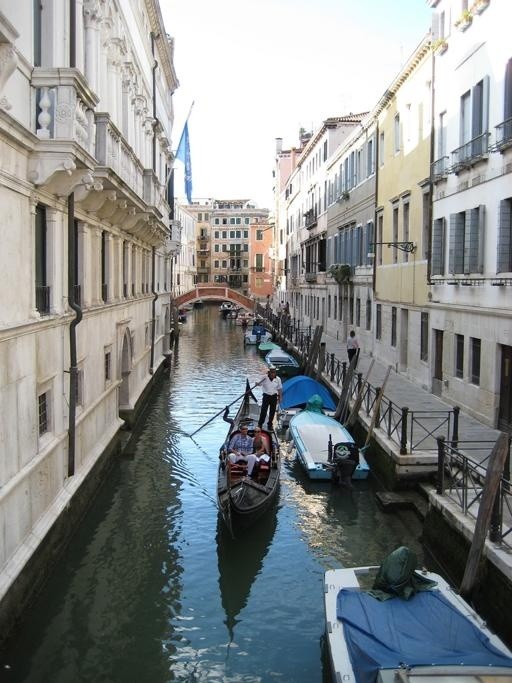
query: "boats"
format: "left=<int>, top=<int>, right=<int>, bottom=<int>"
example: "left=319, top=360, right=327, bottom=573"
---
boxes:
left=273, top=376, right=339, bottom=428
left=321, top=556, right=512, bottom=683
left=177, top=299, right=204, bottom=323
left=216, top=378, right=282, bottom=542
left=286, top=407, right=370, bottom=491
left=219, top=300, right=282, bottom=354
left=211, top=508, right=278, bottom=649
left=265, top=346, right=301, bottom=378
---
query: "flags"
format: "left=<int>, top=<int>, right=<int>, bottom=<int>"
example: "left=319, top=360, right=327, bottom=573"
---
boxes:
left=175, top=120, right=194, bottom=204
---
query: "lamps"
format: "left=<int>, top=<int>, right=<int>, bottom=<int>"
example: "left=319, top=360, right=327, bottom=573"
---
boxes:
left=367, top=241, right=416, bottom=258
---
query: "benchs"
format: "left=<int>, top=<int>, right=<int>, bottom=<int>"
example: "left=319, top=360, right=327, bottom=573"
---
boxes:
left=229, top=430, right=272, bottom=480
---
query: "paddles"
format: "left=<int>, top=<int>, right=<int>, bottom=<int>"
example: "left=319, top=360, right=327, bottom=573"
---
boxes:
left=190, top=378, right=267, bottom=437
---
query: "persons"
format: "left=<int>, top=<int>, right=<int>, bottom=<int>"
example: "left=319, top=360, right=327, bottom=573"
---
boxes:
left=276, top=301, right=289, bottom=317
left=346, top=330, right=358, bottom=367
left=255, top=367, right=282, bottom=431
left=266, top=294, right=273, bottom=308
left=227, top=426, right=271, bottom=480
